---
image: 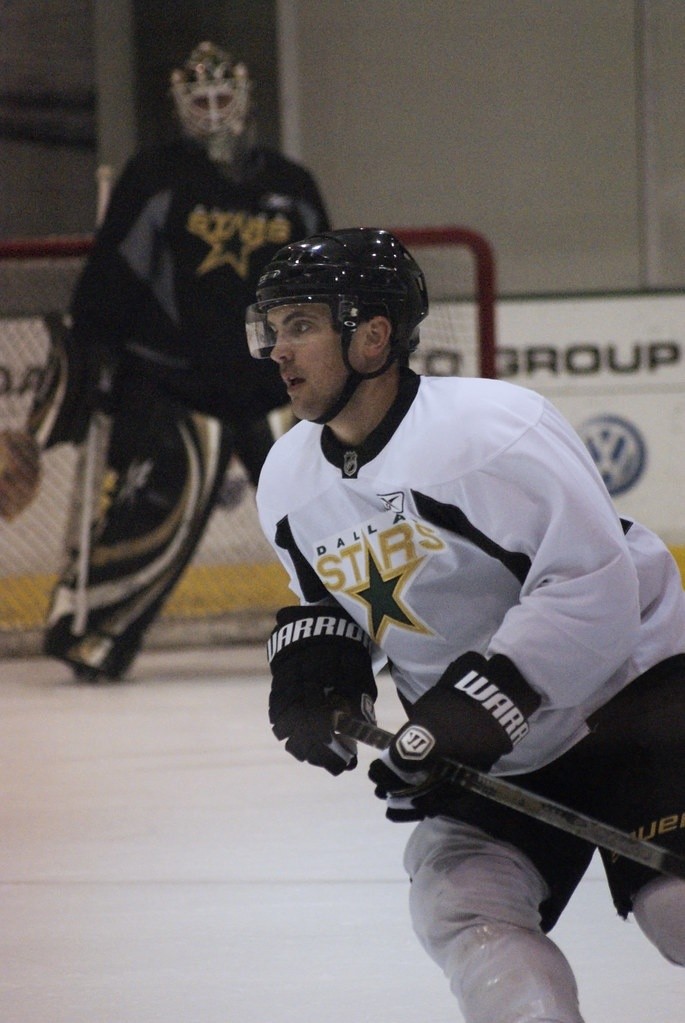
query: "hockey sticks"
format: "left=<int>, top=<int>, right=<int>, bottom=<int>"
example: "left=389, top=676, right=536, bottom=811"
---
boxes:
left=333, top=709, right=684, bottom=883
left=57, top=159, right=127, bottom=677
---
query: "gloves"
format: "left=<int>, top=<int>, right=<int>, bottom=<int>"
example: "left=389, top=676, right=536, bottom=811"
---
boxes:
left=369, top=650, right=542, bottom=823
left=266, top=605, right=379, bottom=776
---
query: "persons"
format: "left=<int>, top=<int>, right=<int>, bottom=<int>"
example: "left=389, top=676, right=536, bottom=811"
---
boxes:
left=253, top=232, right=685, bottom=1023
left=30, top=47, right=340, bottom=679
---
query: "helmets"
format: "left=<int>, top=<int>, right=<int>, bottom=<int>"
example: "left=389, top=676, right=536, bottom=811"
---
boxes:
left=256, top=227, right=430, bottom=354
left=170, top=41, right=248, bottom=142
